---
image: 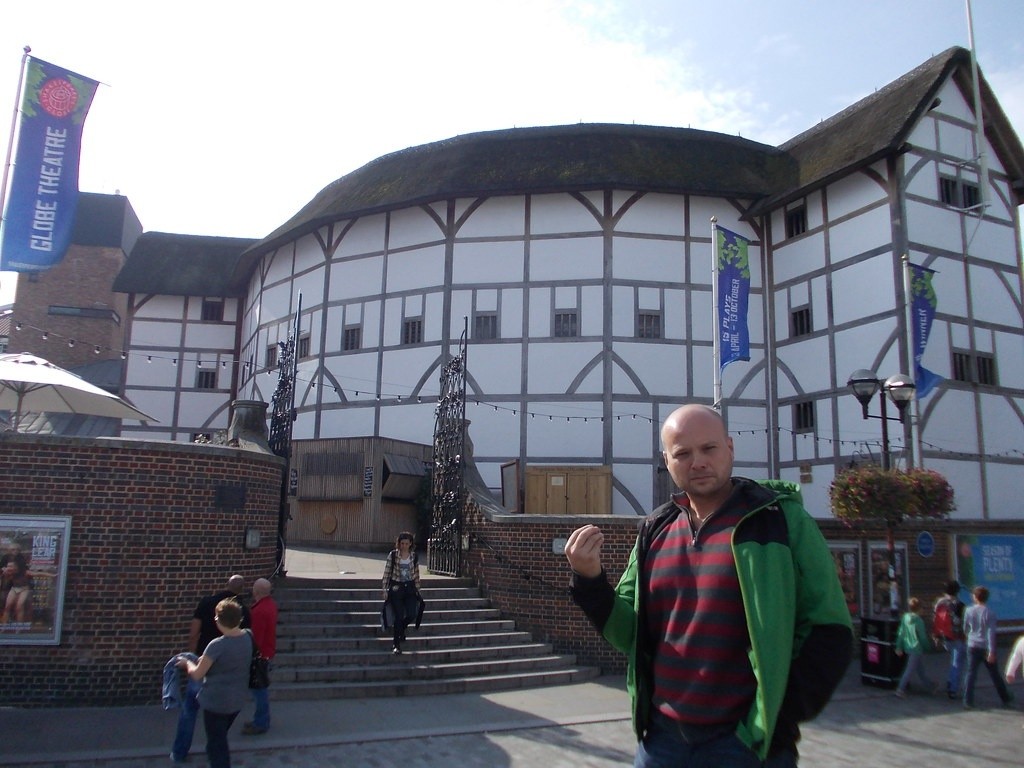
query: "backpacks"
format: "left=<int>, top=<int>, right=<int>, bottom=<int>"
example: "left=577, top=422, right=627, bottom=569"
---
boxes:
left=933, top=600, right=959, bottom=641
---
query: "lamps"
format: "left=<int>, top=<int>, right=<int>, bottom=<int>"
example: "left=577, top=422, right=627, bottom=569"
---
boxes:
left=216, top=486, right=245, bottom=510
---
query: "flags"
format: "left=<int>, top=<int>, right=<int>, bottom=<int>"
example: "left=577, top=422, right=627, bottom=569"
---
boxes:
left=715, top=226, right=752, bottom=376
left=1, top=56, right=101, bottom=275
left=908, top=265, right=945, bottom=400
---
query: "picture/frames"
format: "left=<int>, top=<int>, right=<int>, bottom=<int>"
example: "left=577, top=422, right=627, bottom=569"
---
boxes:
left=0, top=515, right=74, bottom=646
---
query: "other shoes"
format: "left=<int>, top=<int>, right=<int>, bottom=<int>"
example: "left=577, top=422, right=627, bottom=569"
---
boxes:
left=169, top=752, right=174, bottom=761
left=393, top=644, right=402, bottom=655
left=243, top=724, right=268, bottom=734
left=399, top=633, right=406, bottom=640
left=896, top=689, right=908, bottom=700
left=948, top=692, right=956, bottom=700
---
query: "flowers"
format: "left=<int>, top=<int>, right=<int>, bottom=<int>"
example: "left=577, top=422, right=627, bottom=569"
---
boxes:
left=828, top=464, right=956, bottom=529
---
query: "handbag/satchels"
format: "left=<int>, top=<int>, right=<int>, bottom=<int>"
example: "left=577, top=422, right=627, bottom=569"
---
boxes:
left=246, top=629, right=270, bottom=689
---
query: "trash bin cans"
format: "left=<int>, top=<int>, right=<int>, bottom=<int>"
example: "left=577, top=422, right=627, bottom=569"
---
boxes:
left=857, top=614, right=908, bottom=690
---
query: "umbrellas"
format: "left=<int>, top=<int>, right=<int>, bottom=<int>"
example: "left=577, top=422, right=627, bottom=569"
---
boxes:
left=0, top=351, right=163, bottom=432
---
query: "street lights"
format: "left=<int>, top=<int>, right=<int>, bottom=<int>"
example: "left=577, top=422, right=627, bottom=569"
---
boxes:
left=846, top=370, right=918, bottom=617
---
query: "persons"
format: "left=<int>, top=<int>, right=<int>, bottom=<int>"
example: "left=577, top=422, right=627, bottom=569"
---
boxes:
left=895, top=595, right=935, bottom=700
left=961, top=587, right=1015, bottom=710
left=563, top=404, right=854, bottom=768
left=1004, top=635, right=1024, bottom=684
left=170, top=573, right=251, bottom=762
left=0, top=545, right=59, bottom=633
left=241, top=578, right=279, bottom=735
left=381, top=531, right=421, bottom=655
left=174, top=596, right=254, bottom=768
left=928, top=580, right=971, bottom=699
